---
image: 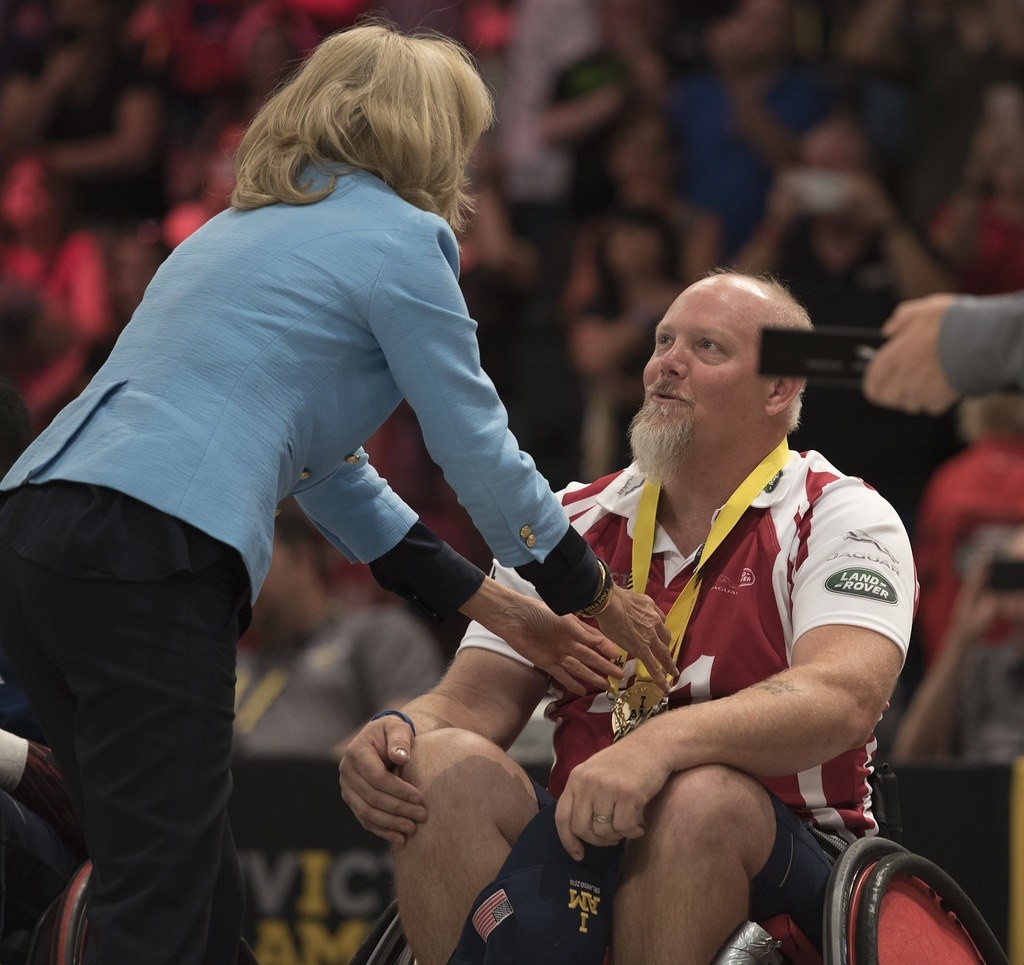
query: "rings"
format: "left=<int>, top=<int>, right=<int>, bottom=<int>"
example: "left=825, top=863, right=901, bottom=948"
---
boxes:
left=591, top=814, right=612, bottom=823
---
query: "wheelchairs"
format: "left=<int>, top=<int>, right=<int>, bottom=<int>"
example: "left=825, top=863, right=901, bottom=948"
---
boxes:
left=343, top=835, right=1012, bottom=965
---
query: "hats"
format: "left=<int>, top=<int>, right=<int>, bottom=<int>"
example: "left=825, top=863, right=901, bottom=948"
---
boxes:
left=444, top=799, right=628, bottom=964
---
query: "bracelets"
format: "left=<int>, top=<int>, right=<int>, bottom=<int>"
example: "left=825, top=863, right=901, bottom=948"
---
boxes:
left=370, top=710, right=416, bottom=737
left=577, top=557, right=612, bottom=618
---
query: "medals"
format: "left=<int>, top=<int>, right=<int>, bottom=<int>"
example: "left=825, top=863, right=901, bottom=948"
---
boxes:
left=612, top=682, right=669, bottom=737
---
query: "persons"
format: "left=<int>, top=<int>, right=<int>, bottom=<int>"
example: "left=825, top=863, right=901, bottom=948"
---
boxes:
left=231, top=501, right=441, bottom=758
left=0, top=27, right=681, bottom=965
left=0, top=0, right=1024, bottom=563
left=864, top=295, right=1024, bottom=412
left=340, top=277, right=923, bottom=965
left=897, top=391, right=1023, bottom=767
